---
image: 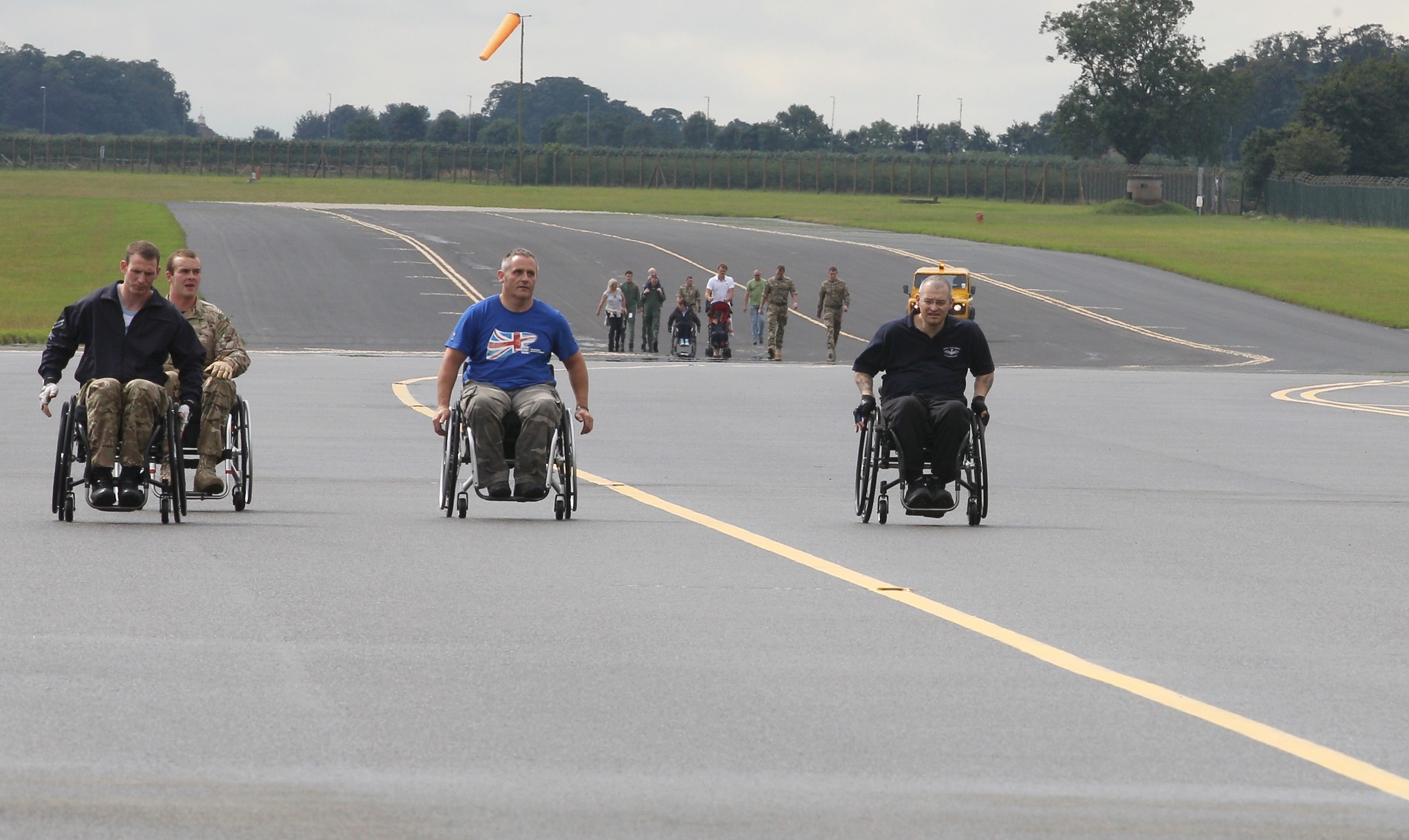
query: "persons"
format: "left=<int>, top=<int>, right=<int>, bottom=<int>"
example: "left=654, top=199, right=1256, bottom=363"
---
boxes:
left=743, top=265, right=798, bottom=360
left=596, top=267, right=666, bottom=352
left=705, top=263, right=735, bottom=357
left=817, top=266, right=850, bottom=361
left=432, top=248, right=594, bottom=497
left=37, top=240, right=251, bottom=508
left=667, top=276, right=701, bottom=346
left=852, top=275, right=995, bottom=519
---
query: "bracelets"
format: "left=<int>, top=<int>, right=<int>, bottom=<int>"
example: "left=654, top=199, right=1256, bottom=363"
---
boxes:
left=575, top=406, right=589, bottom=412
left=698, top=306, right=701, bottom=308
left=794, top=302, right=797, bottom=305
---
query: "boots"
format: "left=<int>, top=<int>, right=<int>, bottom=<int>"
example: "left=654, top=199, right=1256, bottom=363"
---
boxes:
left=775, top=347, right=782, bottom=361
left=767, top=345, right=775, bottom=359
left=193, top=454, right=224, bottom=494
left=117, top=465, right=144, bottom=508
left=827, top=347, right=835, bottom=362
left=90, top=467, right=116, bottom=506
left=159, top=453, right=172, bottom=486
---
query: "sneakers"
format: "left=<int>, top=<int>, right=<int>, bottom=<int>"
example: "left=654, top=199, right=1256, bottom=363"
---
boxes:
left=514, top=482, right=545, bottom=502
left=925, top=489, right=952, bottom=519
left=488, top=482, right=512, bottom=499
left=680, top=339, right=684, bottom=347
left=685, top=339, right=690, bottom=346
left=904, top=488, right=929, bottom=516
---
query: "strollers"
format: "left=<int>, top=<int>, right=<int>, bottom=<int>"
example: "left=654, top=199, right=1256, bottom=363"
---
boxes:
left=705, top=298, right=734, bottom=360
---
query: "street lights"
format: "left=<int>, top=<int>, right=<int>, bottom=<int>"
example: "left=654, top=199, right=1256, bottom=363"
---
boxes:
left=703, top=96, right=710, bottom=150
left=466, top=95, right=472, bottom=145
left=584, top=94, right=590, bottom=150
left=829, top=96, right=836, bottom=154
left=915, top=94, right=922, bottom=154
left=958, top=97, right=963, bottom=153
left=327, top=92, right=332, bottom=139
left=40, top=86, right=47, bottom=137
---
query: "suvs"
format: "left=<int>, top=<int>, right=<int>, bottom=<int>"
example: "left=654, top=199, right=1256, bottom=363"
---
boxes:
left=903, top=263, right=977, bottom=321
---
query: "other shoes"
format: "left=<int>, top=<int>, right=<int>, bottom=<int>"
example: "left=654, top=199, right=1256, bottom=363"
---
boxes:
left=753, top=340, right=757, bottom=345
left=713, top=349, right=719, bottom=358
left=620, top=341, right=659, bottom=352
left=719, top=349, right=724, bottom=356
left=826, top=305, right=842, bottom=309
left=759, top=338, right=763, bottom=343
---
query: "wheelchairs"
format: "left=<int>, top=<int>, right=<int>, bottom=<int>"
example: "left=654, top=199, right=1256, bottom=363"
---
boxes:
left=148, top=394, right=254, bottom=512
left=669, top=322, right=698, bottom=360
left=853, top=387, right=990, bottom=527
left=51, top=393, right=188, bottom=524
left=438, top=361, right=577, bottom=520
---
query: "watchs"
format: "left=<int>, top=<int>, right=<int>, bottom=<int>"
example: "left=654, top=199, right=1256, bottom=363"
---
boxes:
left=42, top=378, right=57, bottom=385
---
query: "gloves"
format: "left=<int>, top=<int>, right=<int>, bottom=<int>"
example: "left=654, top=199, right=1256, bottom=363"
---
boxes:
left=203, top=357, right=234, bottom=380
left=667, top=326, right=672, bottom=333
left=696, top=326, right=700, bottom=333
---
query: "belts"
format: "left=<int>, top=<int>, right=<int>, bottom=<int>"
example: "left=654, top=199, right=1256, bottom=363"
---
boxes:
left=771, top=301, right=787, bottom=307
